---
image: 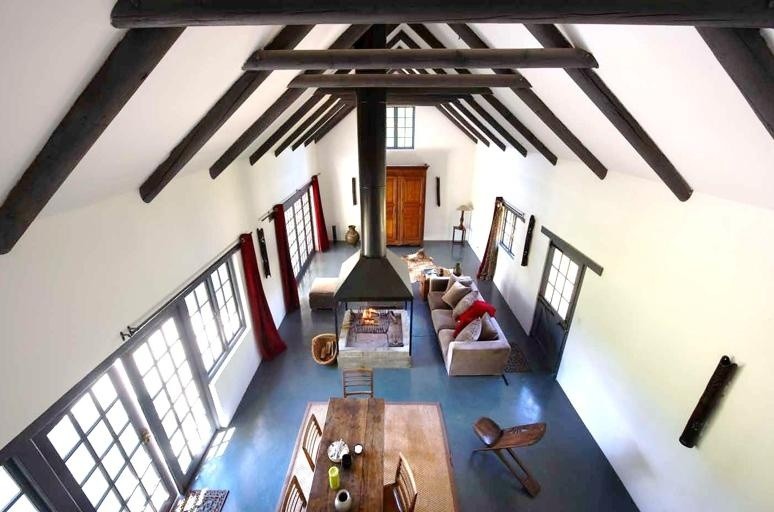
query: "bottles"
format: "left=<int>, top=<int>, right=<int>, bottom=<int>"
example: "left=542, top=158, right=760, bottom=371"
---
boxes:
left=328, top=466, right=340, bottom=489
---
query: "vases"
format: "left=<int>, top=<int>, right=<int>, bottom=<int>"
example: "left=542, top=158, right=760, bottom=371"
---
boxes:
left=345, top=225, right=359, bottom=245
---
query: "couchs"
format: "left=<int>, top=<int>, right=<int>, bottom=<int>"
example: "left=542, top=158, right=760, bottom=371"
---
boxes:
left=429, top=275, right=511, bottom=376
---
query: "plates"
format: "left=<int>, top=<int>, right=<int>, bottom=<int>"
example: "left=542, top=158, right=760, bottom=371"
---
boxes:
left=326, top=441, right=350, bottom=462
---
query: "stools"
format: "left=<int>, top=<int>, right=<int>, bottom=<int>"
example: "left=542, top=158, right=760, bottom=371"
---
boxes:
left=309, top=277, right=341, bottom=313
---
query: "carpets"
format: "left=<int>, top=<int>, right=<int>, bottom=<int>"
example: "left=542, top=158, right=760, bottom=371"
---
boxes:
left=275, top=401, right=459, bottom=512
left=401, top=248, right=434, bottom=283
left=506, top=342, right=531, bottom=372
left=169, top=489, right=230, bottom=512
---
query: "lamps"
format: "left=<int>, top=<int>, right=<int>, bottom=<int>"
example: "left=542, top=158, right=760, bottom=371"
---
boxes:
left=456, top=205, right=471, bottom=228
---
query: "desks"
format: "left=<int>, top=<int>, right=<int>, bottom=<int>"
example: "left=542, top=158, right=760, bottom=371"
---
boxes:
left=453, top=226, right=466, bottom=245
left=307, top=397, right=385, bottom=512
left=420, top=268, right=455, bottom=301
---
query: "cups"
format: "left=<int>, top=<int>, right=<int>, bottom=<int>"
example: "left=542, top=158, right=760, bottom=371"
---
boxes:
left=342, top=453, right=353, bottom=471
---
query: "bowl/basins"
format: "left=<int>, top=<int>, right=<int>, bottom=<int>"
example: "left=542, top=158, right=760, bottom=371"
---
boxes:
left=353, top=443, right=363, bottom=455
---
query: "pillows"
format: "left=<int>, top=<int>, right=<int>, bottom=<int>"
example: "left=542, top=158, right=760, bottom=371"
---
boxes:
left=443, top=274, right=499, bottom=342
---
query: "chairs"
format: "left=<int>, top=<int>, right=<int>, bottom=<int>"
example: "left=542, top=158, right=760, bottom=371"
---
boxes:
left=383, top=453, right=419, bottom=512
left=342, top=368, right=374, bottom=397
left=280, top=475, right=315, bottom=512
left=303, top=412, right=322, bottom=471
left=472, top=416, right=546, bottom=497
